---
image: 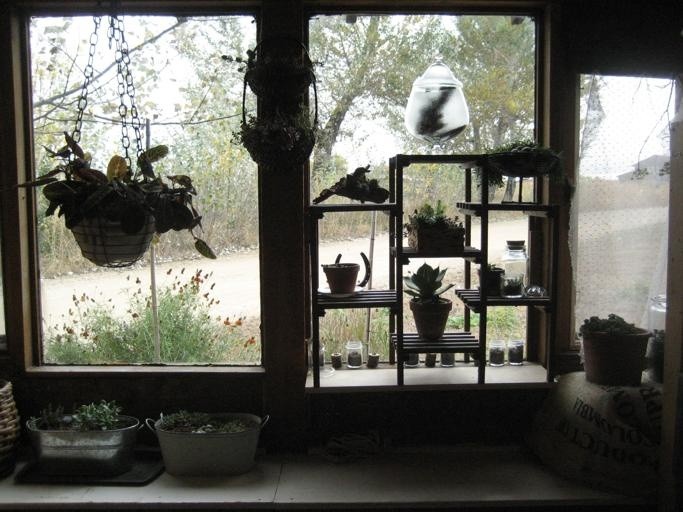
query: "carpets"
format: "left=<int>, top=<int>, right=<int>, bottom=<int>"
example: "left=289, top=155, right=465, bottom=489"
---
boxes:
left=14, top=448, right=165, bottom=487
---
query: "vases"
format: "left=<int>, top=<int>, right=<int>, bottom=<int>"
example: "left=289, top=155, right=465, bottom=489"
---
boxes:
left=323, top=263, right=360, bottom=293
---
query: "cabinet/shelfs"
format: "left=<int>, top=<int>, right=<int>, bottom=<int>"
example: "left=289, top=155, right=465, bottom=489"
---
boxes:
left=309, top=153, right=560, bottom=388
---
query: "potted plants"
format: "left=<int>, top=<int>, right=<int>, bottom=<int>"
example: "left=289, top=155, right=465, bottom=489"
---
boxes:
left=390, top=200, right=505, bottom=341
left=10, top=127, right=216, bottom=270
left=577, top=312, right=665, bottom=386
left=24, top=398, right=269, bottom=478
left=473, top=138, right=562, bottom=203
left=221, top=33, right=324, bottom=169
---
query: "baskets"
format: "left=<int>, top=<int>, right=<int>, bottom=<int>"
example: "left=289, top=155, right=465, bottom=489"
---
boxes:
left=0, top=379, right=22, bottom=477
left=61, top=199, right=159, bottom=268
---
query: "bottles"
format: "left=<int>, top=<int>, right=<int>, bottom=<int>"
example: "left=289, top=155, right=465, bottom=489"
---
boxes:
left=346, top=339, right=363, bottom=369
left=501, top=239, right=531, bottom=298
left=489, top=338, right=506, bottom=367
left=307, top=337, right=325, bottom=370
left=508, top=339, right=524, bottom=366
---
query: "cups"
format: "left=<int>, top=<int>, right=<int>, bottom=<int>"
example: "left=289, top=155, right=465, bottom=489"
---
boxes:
left=500, top=273, right=525, bottom=299
left=404, top=353, right=420, bottom=367
left=440, top=353, right=455, bottom=367
